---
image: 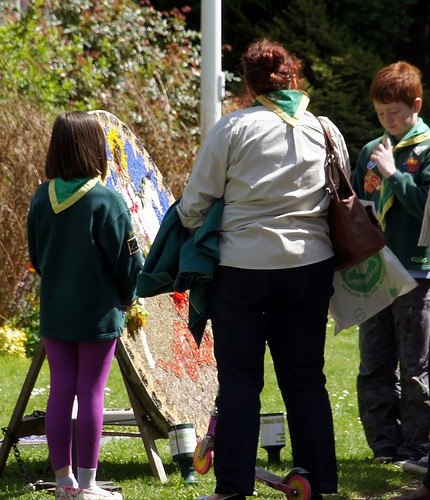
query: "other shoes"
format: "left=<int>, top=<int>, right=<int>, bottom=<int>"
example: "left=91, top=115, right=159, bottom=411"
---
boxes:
left=198, top=494, right=246, bottom=500
left=371, top=446, right=396, bottom=464
left=403, top=457, right=428, bottom=475
left=54, top=486, right=81, bottom=500
left=77, top=486, right=123, bottom=500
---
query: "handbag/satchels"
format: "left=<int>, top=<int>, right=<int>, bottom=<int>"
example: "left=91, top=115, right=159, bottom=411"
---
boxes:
left=329, top=200, right=418, bottom=336
left=316, top=116, right=387, bottom=267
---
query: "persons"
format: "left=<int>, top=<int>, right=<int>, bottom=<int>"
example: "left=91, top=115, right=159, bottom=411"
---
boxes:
left=176, top=40, right=351, bottom=500
left=26, top=111, right=144, bottom=500
left=352, top=61, right=430, bottom=478
